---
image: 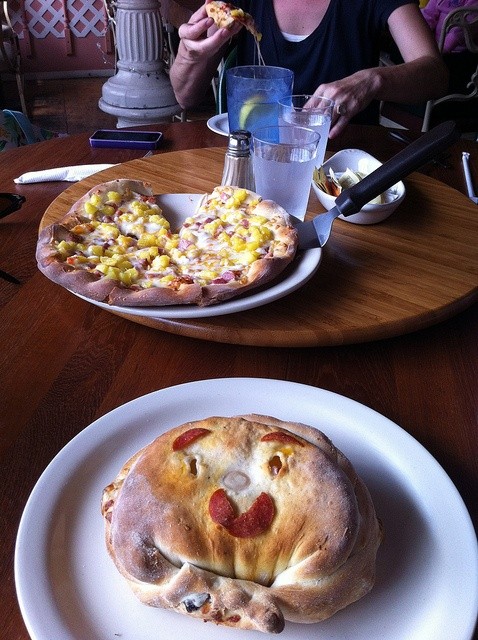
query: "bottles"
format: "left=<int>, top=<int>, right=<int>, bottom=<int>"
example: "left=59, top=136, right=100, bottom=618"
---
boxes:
left=222, top=129, right=254, bottom=190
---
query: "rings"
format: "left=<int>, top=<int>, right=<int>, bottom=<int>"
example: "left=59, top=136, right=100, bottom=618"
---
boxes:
left=328, top=102, right=349, bottom=117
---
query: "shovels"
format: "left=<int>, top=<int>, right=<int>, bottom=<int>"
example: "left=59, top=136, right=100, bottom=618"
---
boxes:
left=294, top=120, right=459, bottom=252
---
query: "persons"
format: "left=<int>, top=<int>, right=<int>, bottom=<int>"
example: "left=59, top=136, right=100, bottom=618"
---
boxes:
left=168, top=0, right=446, bottom=142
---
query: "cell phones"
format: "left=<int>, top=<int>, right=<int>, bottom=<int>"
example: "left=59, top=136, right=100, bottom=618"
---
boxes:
left=89, top=127, right=164, bottom=150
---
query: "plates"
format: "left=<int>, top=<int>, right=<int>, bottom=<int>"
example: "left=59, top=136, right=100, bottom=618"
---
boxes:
left=12, top=370, right=478, bottom=640
left=206, top=111, right=229, bottom=139
left=62, top=190, right=326, bottom=318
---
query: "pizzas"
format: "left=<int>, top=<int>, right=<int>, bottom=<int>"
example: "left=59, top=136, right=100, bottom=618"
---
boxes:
left=36, top=179, right=298, bottom=307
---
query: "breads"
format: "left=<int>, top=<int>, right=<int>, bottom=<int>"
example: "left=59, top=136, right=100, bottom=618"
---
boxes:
left=101, top=413, right=383, bottom=633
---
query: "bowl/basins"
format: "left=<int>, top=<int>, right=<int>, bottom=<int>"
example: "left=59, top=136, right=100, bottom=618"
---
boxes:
left=309, top=144, right=406, bottom=226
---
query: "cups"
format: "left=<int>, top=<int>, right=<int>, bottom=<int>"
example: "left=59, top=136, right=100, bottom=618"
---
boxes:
left=275, top=95, right=334, bottom=170
left=251, top=125, right=319, bottom=220
left=227, top=66, right=294, bottom=144
